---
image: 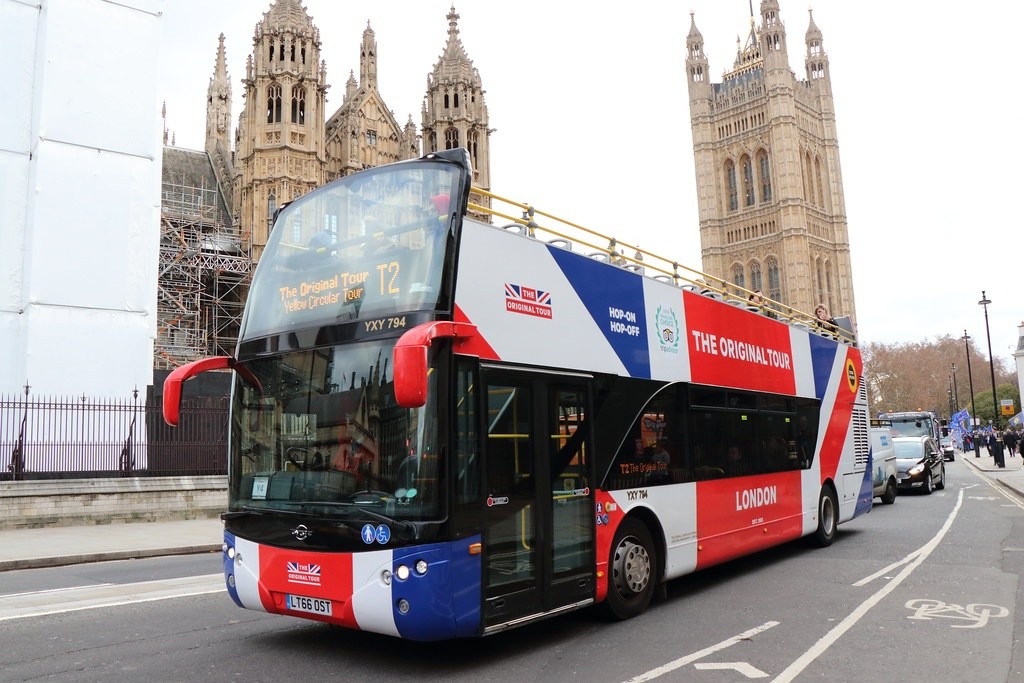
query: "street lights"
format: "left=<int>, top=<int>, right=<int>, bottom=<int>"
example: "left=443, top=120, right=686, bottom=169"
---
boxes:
left=978, top=291, right=1011, bottom=468
left=951, top=362, right=959, bottom=412
left=948, top=375, right=954, bottom=415
left=962, top=329, right=981, bottom=458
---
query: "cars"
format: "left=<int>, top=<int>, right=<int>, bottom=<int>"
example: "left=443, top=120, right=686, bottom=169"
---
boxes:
left=936, top=432, right=958, bottom=461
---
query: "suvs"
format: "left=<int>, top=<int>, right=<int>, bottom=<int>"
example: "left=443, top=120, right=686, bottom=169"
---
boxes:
left=891, top=434, right=945, bottom=494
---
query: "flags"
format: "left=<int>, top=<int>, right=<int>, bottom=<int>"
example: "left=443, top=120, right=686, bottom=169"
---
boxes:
left=1008, top=411, right=1024, bottom=427
left=948, top=409, right=971, bottom=430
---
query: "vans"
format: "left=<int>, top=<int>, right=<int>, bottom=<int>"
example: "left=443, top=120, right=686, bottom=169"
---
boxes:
left=870, top=428, right=898, bottom=504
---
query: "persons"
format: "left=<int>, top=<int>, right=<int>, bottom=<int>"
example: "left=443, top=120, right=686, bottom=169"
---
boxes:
left=285, top=230, right=352, bottom=270
left=691, top=278, right=716, bottom=299
left=330, top=408, right=379, bottom=475
left=361, top=217, right=401, bottom=257
left=723, top=445, right=760, bottom=478
left=964, top=426, right=1024, bottom=466
left=812, top=304, right=840, bottom=341
left=746, top=290, right=777, bottom=319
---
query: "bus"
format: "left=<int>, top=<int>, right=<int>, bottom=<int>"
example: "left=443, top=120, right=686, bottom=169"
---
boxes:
left=878, top=408, right=948, bottom=450
left=559, top=413, right=664, bottom=466
left=162, top=148, right=874, bottom=642
left=692, top=405, right=807, bottom=460
